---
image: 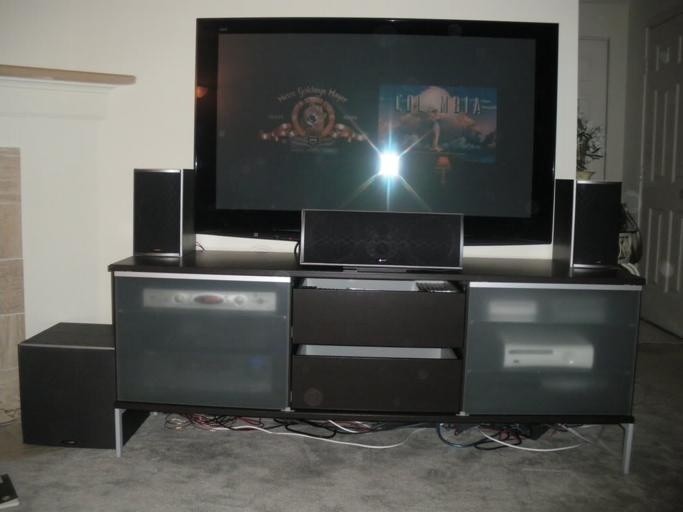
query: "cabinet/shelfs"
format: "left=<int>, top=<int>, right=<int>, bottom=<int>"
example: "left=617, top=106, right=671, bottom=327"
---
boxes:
left=113, top=273, right=642, bottom=476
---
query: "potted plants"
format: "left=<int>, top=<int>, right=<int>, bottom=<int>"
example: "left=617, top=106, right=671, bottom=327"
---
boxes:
left=578, top=112, right=604, bottom=179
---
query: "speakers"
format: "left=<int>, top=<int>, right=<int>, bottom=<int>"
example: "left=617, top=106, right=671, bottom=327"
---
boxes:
left=300, top=207, right=463, bottom=271
left=553, top=178, right=622, bottom=268
left=133, top=169, right=196, bottom=258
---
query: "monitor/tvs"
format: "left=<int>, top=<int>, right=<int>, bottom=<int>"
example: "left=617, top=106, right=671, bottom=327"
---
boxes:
left=194, top=16, right=558, bottom=244
